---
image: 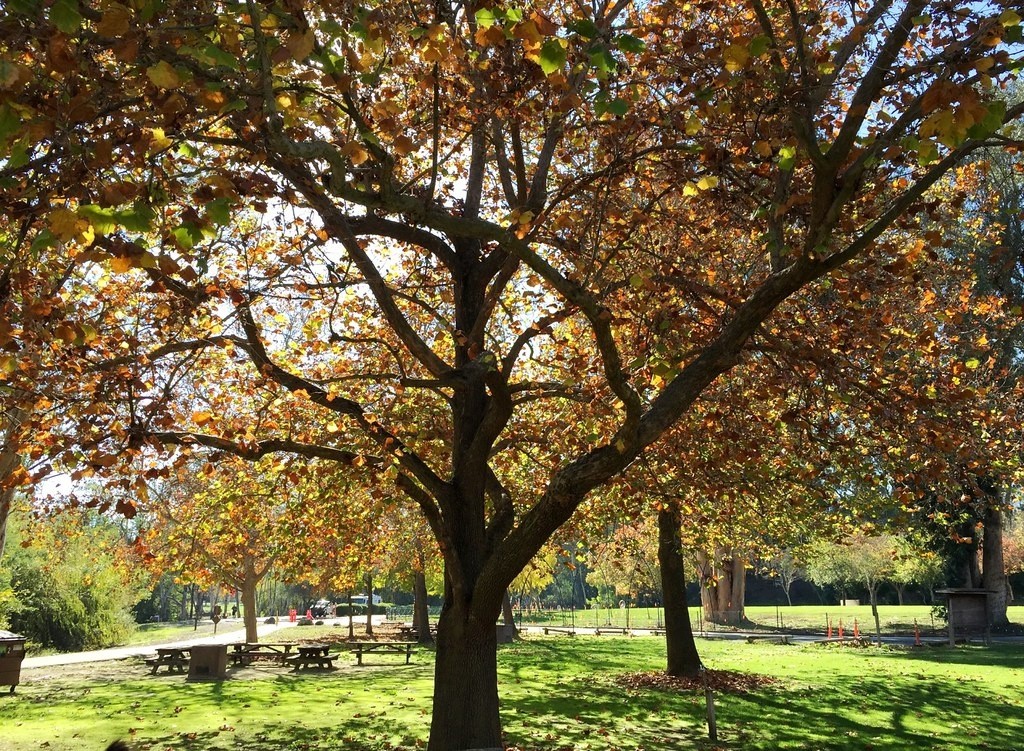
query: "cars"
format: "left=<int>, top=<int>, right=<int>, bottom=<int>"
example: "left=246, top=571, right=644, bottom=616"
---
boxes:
left=309, top=600, right=333, bottom=618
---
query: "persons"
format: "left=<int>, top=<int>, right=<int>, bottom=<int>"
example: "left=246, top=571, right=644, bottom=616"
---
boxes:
left=310, top=601, right=336, bottom=618
left=231, top=604, right=238, bottom=616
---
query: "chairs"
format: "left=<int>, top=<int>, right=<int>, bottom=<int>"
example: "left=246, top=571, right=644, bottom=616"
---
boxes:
left=144, top=641, right=340, bottom=672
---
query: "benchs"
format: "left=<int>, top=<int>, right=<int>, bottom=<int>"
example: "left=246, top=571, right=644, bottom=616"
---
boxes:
left=348, top=649, right=419, bottom=666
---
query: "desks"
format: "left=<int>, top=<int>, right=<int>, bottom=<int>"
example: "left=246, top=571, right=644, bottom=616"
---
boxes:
left=348, top=641, right=419, bottom=667
